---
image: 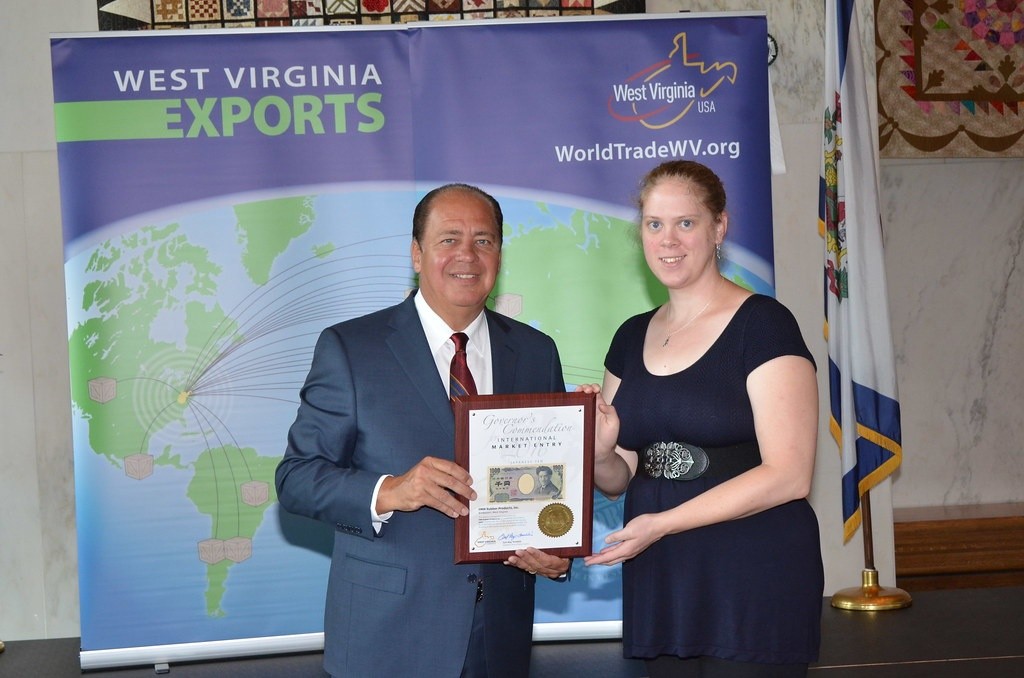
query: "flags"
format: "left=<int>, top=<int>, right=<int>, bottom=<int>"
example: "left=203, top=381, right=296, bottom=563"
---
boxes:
left=817, top=0, right=902, bottom=546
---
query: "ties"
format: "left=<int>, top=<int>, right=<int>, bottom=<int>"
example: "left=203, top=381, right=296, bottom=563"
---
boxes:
left=449, top=333, right=478, bottom=416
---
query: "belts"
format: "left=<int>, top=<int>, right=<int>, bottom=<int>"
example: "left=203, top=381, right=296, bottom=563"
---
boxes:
left=636, top=441, right=761, bottom=481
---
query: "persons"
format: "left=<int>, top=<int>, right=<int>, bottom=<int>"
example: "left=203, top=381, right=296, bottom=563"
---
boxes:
left=274, top=159, right=820, bottom=678
